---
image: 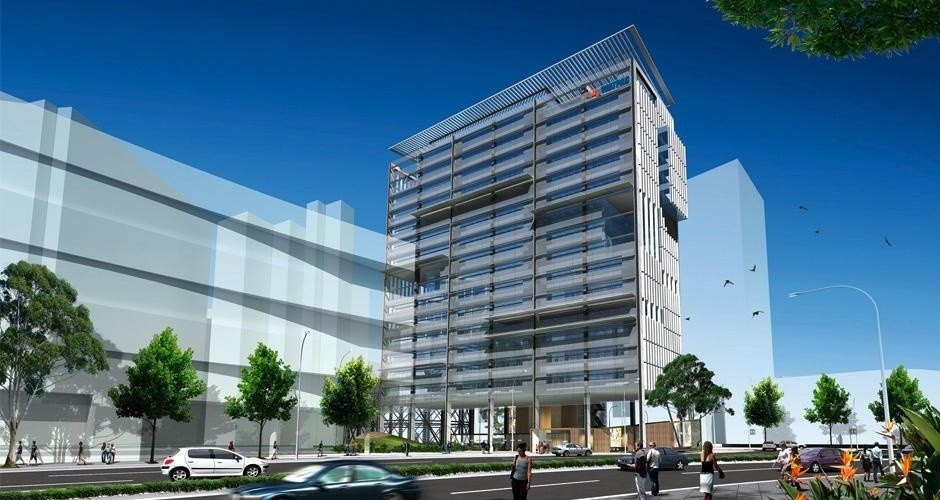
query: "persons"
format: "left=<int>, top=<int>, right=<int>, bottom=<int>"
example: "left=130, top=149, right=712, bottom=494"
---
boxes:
left=405, top=441, right=409, bottom=456
left=536, top=440, right=550, bottom=454
left=76, top=441, right=86, bottom=465
left=344, top=437, right=359, bottom=456
left=317, top=440, right=323, bottom=457
left=442, top=441, right=451, bottom=454
left=870, top=442, right=885, bottom=483
left=401, top=442, right=405, bottom=453
left=511, top=442, right=532, bottom=500
left=646, top=442, right=662, bottom=496
left=29, top=441, right=38, bottom=464
left=860, top=444, right=873, bottom=482
left=481, top=440, right=486, bottom=454
left=228, top=441, right=234, bottom=451
left=15, top=441, right=24, bottom=464
left=697, top=441, right=702, bottom=448
left=271, top=441, right=280, bottom=460
left=626, top=441, right=648, bottom=500
left=699, top=441, right=725, bottom=500
left=772, top=442, right=791, bottom=488
left=789, top=446, right=802, bottom=492
left=101, top=442, right=115, bottom=463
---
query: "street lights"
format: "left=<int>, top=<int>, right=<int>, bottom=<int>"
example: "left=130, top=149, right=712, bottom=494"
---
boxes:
left=294, top=328, right=312, bottom=461
left=608, top=405, right=618, bottom=427
left=839, top=385, right=859, bottom=451
left=788, top=284, right=897, bottom=476
left=510, top=368, right=528, bottom=453
left=623, top=378, right=640, bottom=454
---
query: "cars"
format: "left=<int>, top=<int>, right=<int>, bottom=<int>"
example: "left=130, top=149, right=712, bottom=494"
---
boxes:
left=776, top=439, right=807, bottom=449
left=550, top=441, right=594, bottom=457
left=795, top=447, right=847, bottom=474
left=160, top=445, right=273, bottom=482
left=617, top=445, right=689, bottom=472
left=900, top=443, right=914, bottom=454
left=227, top=457, right=423, bottom=500
left=762, top=441, right=777, bottom=451
